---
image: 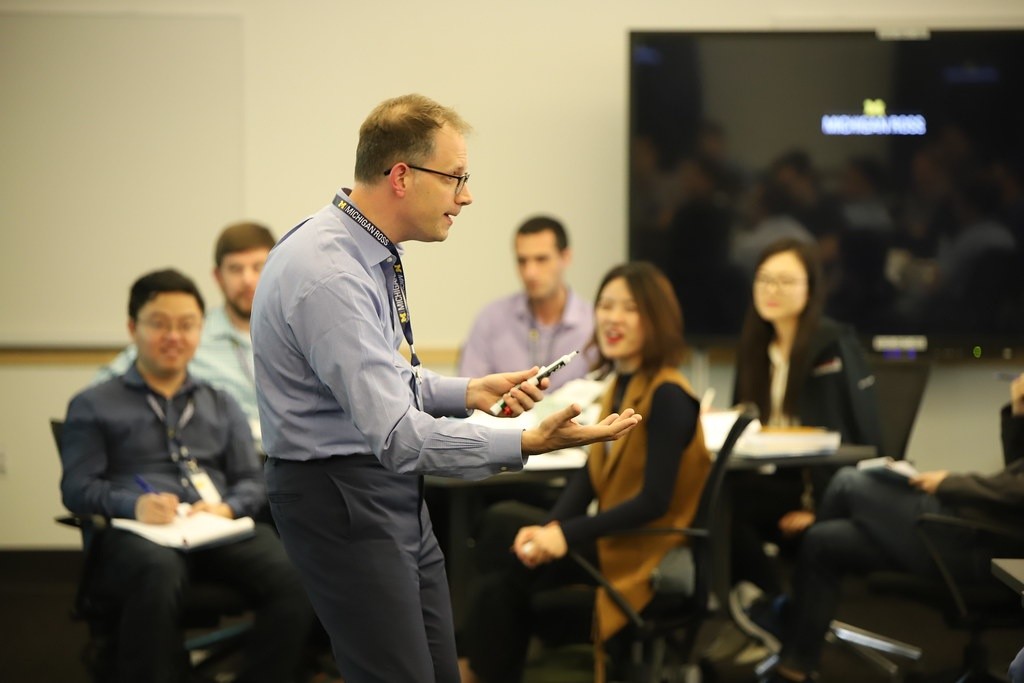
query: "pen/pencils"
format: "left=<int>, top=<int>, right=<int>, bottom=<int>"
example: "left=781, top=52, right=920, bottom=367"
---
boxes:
left=509, top=520, right=559, bottom=550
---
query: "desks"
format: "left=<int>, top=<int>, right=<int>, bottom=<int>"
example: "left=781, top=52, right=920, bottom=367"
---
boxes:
left=423, top=443, right=875, bottom=622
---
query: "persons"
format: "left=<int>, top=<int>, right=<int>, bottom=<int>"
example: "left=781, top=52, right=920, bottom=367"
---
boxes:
left=458, top=262, right=714, bottom=683
left=59, top=225, right=310, bottom=683
left=705, top=237, right=891, bottom=665
left=460, top=218, right=615, bottom=394
left=631, top=118, right=1023, bottom=336
left=729, top=375, right=1024, bottom=683
left=250, top=95, right=642, bottom=683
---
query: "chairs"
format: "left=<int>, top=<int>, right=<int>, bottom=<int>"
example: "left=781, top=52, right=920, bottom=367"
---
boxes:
left=49, top=339, right=1024, bottom=683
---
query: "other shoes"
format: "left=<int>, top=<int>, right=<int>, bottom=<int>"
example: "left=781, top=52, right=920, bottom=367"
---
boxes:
left=700, top=658, right=813, bottom=683
left=726, top=578, right=784, bottom=651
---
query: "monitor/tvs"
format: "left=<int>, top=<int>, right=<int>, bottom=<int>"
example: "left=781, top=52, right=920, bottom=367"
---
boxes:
left=628, top=28, right=1024, bottom=364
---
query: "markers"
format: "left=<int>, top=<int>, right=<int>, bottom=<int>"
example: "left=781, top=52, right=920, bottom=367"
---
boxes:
left=489, top=350, right=580, bottom=415
left=503, top=366, right=547, bottom=415
left=134, top=475, right=181, bottom=517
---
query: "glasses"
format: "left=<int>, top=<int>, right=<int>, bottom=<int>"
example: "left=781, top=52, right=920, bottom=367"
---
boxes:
left=384, top=164, right=470, bottom=195
